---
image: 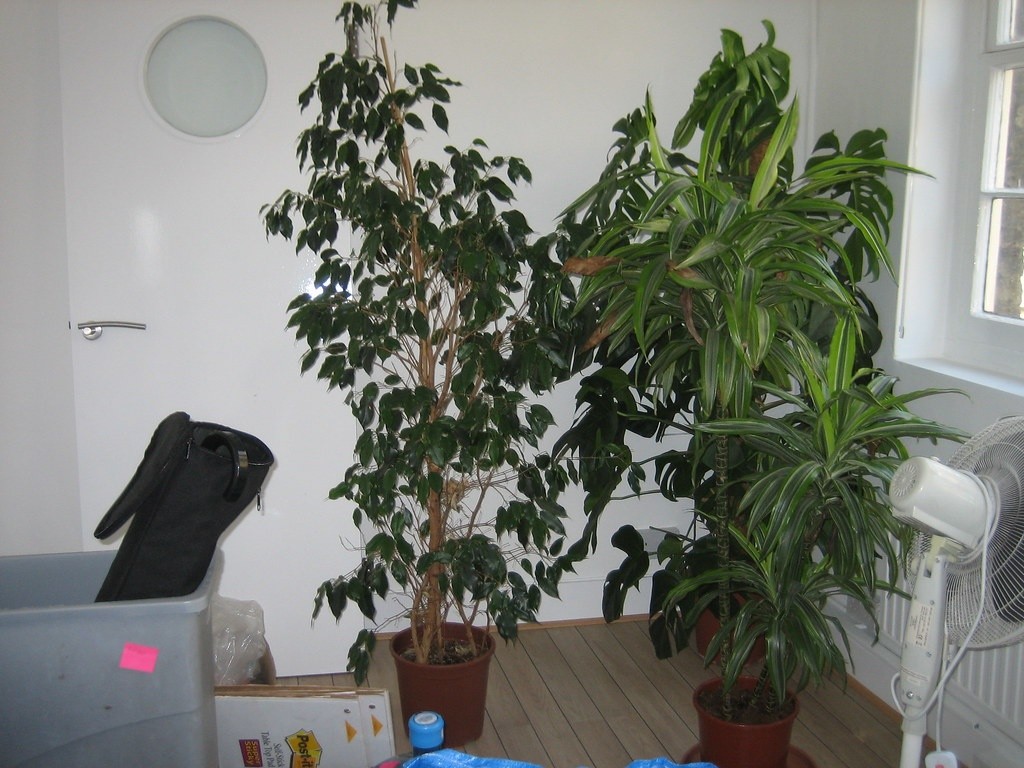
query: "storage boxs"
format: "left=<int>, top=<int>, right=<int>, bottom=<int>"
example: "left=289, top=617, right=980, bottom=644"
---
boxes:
left=0, top=549, right=220, bottom=768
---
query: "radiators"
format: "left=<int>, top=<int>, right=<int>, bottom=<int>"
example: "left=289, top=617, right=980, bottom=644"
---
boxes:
left=844, top=473, right=1024, bottom=748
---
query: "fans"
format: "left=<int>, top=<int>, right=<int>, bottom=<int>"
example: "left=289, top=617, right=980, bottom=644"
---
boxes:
left=888, top=413, right=1024, bottom=768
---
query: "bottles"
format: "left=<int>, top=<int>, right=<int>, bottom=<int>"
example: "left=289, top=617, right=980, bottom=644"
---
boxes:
left=370, top=711, right=445, bottom=768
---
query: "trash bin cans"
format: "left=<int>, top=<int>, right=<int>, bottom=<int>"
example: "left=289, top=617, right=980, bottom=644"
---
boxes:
left=0, top=544, right=224, bottom=768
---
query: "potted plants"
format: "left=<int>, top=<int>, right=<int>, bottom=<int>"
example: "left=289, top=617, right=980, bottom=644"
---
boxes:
left=258, top=0, right=578, bottom=751
left=555, top=18, right=971, bottom=768
